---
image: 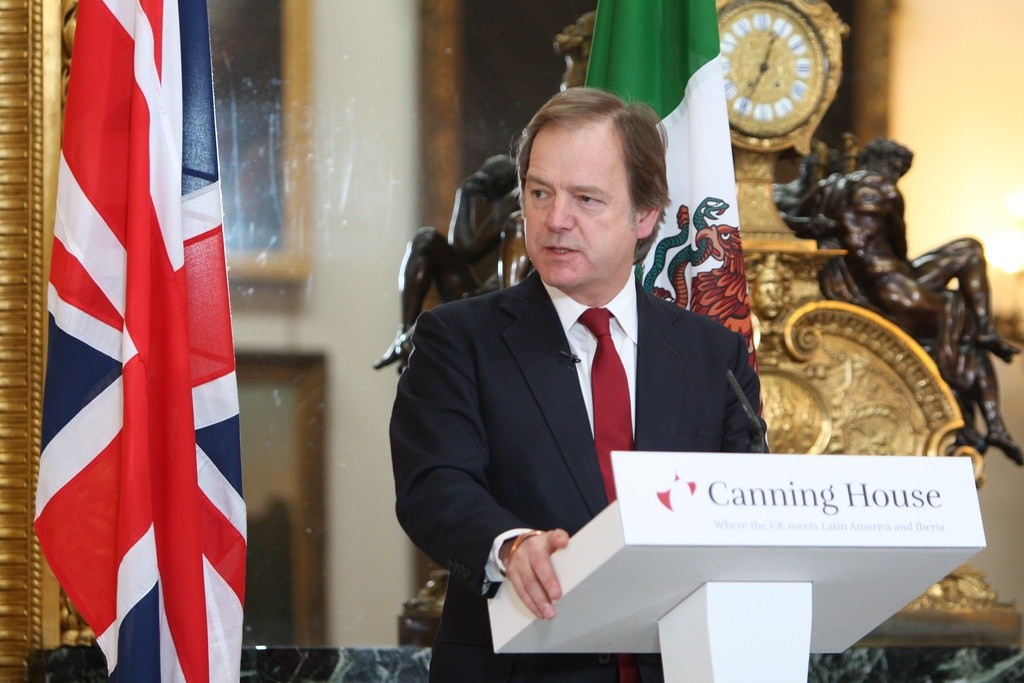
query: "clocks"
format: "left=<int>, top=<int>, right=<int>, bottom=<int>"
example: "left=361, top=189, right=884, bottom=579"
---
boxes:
left=716, top=0, right=851, bottom=155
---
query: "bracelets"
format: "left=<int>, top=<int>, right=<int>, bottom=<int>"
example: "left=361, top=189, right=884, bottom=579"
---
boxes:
left=504, top=530, right=542, bottom=564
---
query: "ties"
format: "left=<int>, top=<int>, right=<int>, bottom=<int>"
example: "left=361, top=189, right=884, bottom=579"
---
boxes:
left=577, top=307, right=634, bottom=502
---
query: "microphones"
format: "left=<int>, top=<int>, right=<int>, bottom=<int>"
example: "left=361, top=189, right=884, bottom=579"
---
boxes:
left=559, top=350, right=581, bottom=362
left=726, top=369, right=764, bottom=453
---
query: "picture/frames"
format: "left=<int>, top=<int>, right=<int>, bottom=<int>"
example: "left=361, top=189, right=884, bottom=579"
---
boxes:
left=234, top=346, right=330, bottom=646
left=420, top=1, right=897, bottom=308
left=209, top=1, right=315, bottom=282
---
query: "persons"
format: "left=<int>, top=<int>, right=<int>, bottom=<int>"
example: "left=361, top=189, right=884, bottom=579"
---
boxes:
left=390, top=86, right=769, bottom=682
left=373, top=11, right=597, bottom=369
left=789, top=139, right=1024, bottom=466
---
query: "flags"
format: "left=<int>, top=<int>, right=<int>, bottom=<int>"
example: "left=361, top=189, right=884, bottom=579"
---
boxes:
left=585, top=0, right=764, bottom=413
left=36, top=0, right=245, bottom=683
